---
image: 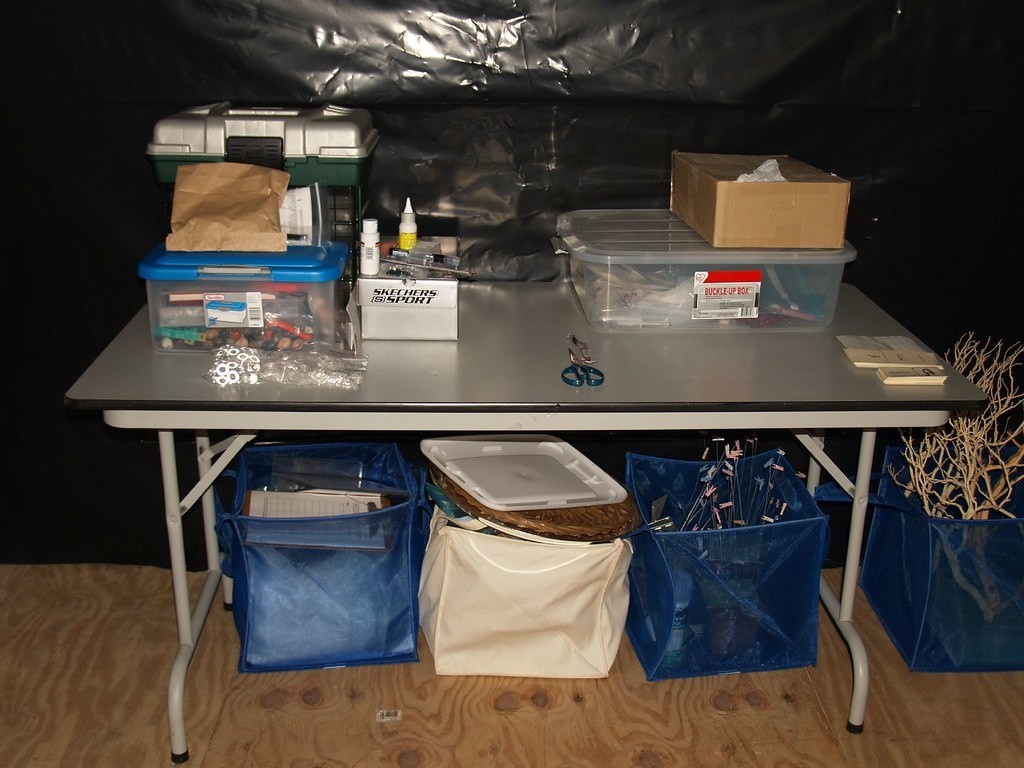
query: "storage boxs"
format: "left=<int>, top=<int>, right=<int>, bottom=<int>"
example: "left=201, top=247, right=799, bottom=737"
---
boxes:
left=354, top=235, right=461, bottom=340
left=145, top=100, right=380, bottom=217
left=139, top=240, right=350, bottom=355
left=550, top=209, right=857, bottom=331
left=670, top=149, right=851, bottom=249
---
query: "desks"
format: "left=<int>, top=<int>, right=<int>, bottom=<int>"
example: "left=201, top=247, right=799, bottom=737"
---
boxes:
left=61, top=281, right=989, bottom=764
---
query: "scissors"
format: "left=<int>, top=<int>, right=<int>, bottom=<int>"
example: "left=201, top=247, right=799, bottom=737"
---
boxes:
left=561, top=348, right=605, bottom=387
